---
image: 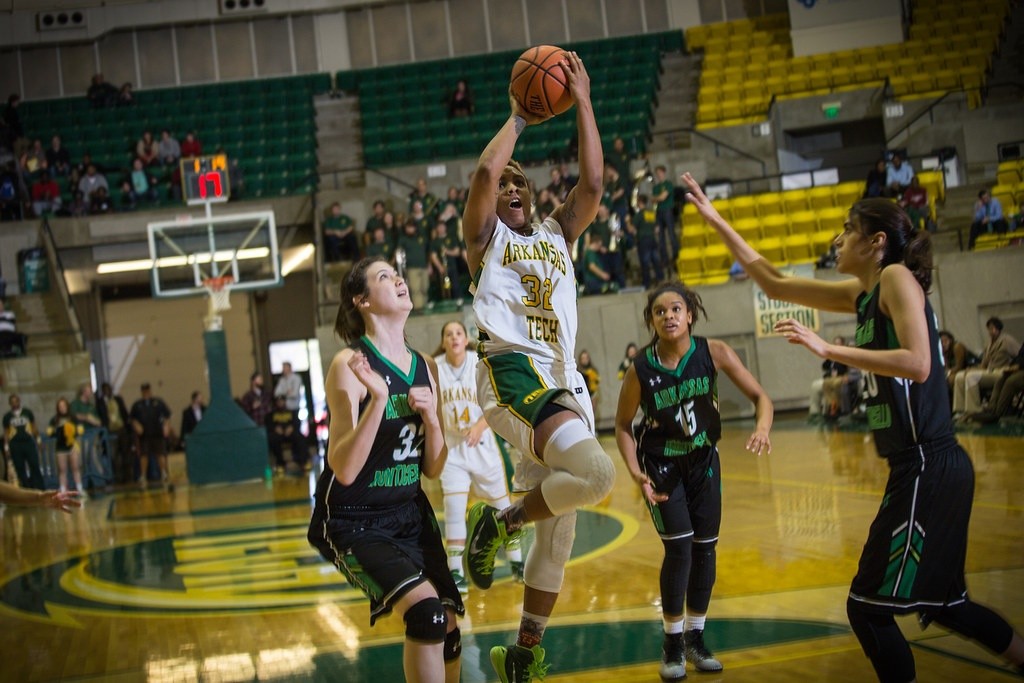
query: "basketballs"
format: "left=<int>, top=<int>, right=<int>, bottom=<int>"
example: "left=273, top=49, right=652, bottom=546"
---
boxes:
left=511, top=43, right=576, bottom=117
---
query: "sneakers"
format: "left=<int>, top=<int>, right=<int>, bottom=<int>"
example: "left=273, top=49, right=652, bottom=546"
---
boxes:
left=450, top=568, right=470, bottom=595
left=683, top=629, right=722, bottom=673
left=513, top=566, right=525, bottom=583
left=462, top=500, right=524, bottom=591
left=660, top=637, right=686, bottom=683
left=489, top=643, right=550, bottom=683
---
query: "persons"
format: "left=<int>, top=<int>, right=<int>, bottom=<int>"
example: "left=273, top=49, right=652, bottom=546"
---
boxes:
left=616, top=278, right=773, bottom=681
left=0, top=481, right=82, bottom=516
left=0, top=70, right=1024, bottom=497
left=461, top=52, right=615, bottom=683
left=307, top=255, right=466, bottom=683
left=682, top=171, right=1024, bottom=683
left=433, top=320, right=526, bottom=593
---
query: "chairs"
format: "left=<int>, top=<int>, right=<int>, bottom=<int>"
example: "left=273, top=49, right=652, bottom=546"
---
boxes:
left=0, top=0, right=1024, bottom=313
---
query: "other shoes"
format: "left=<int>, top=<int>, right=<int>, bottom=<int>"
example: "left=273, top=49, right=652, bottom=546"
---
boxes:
left=971, top=410, right=999, bottom=426
